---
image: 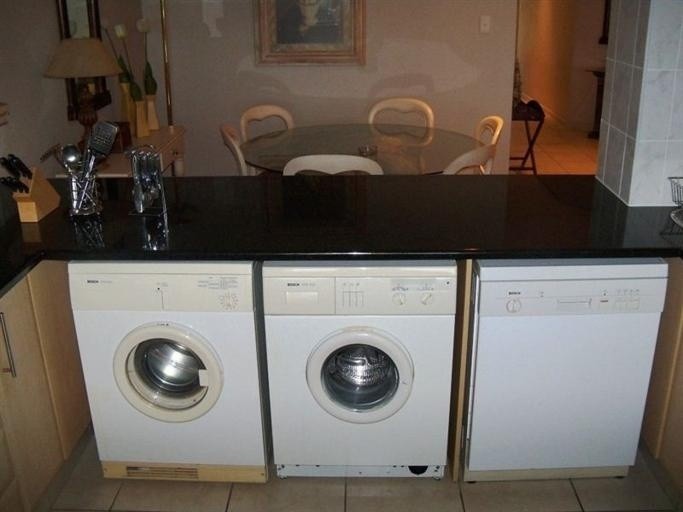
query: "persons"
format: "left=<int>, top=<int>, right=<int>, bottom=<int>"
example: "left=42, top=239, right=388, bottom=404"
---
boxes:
left=277, top=0, right=343, bottom=44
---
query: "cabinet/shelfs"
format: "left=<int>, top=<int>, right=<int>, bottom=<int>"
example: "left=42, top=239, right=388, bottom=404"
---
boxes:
left=640, top=257, right=683, bottom=501
left=511, top=63, right=543, bottom=176
left=1, top=259, right=89, bottom=511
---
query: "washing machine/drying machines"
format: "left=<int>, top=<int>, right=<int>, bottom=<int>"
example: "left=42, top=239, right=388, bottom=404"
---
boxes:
left=64, top=260, right=274, bottom=483
left=260, top=261, right=458, bottom=483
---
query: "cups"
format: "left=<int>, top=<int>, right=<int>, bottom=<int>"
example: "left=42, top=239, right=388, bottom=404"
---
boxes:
left=71, top=218, right=106, bottom=254
left=63, top=170, right=102, bottom=217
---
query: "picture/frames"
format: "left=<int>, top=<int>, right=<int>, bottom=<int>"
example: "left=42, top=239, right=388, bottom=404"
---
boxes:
left=250, top=1, right=366, bottom=67
left=56, top=0, right=113, bottom=122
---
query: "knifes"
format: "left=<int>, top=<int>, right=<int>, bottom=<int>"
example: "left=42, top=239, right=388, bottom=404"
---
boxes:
left=0, top=152, right=32, bottom=195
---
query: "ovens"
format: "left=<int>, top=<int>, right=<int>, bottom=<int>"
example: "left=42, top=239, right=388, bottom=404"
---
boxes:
left=460, top=257, right=674, bottom=485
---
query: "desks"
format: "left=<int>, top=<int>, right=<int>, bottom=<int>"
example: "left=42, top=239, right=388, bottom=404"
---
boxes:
left=54, top=124, right=193, bottom=179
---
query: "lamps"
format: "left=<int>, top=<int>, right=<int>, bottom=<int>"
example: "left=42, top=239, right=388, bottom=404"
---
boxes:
left=43, top=38, right=123, bottom=155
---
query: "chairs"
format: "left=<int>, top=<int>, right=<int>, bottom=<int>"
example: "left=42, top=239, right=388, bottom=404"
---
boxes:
left=219, top=96, right=502, bottom=175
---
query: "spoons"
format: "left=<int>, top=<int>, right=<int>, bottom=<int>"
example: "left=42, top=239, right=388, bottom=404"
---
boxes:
left=128, top=153, right=163, bottom=214
left=60, top=142, right=83, bottom=173
